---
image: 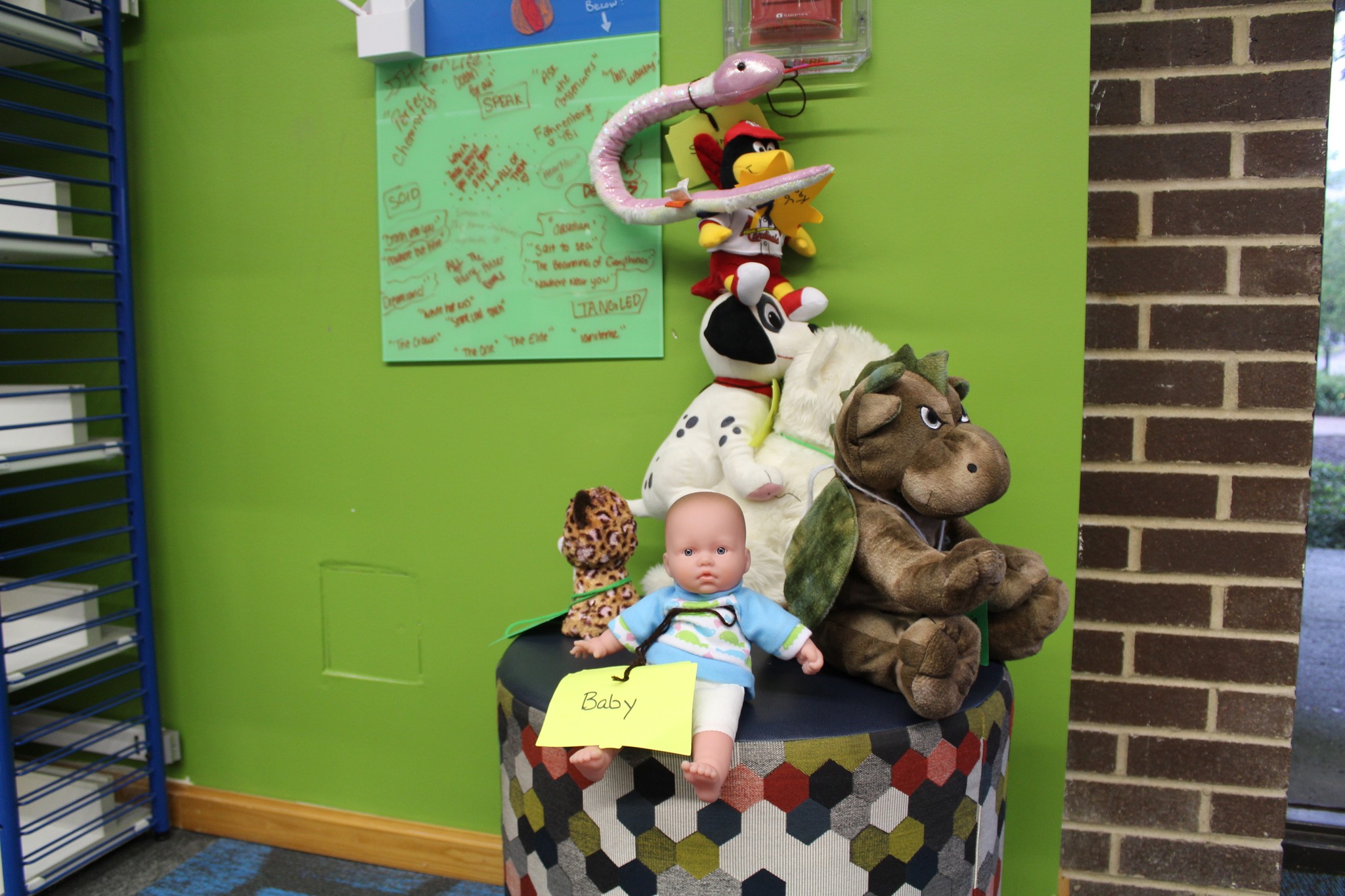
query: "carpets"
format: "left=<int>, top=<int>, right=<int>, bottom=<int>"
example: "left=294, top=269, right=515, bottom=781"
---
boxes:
left=135, top=837, right=505, bottom=896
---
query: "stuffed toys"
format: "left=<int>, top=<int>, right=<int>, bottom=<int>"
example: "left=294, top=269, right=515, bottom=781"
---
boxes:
left=691, top=121, right=834, bottom=323
left=642, top=323, right=895, bottom=609
left=625, top=288, right=823, bottom=517
left=558, top=486, right=637, bottom=639
left=588, top=51, right=843, bottom=226
left=783, top=343, right=1071, bottom=717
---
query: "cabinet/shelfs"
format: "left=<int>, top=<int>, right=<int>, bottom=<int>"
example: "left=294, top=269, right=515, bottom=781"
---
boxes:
left=0, top=0, right=172, bottom=895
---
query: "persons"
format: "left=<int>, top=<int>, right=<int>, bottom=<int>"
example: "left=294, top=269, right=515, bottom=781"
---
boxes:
left=569, top=491, right=824, bottom=802
